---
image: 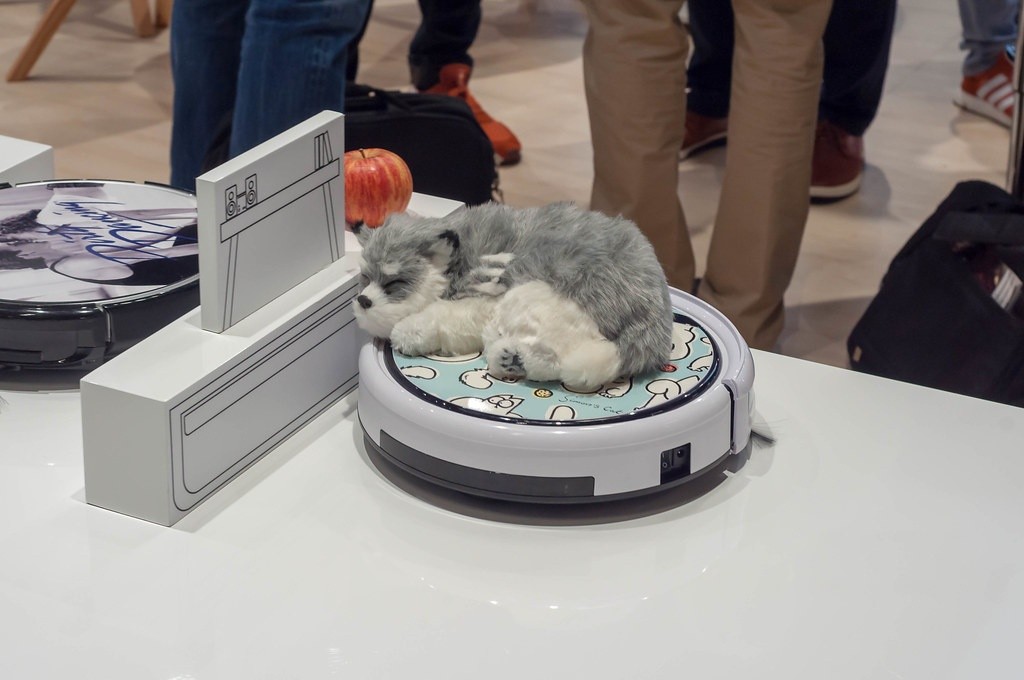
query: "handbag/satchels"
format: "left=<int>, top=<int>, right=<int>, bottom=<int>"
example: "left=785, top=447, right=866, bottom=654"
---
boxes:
left=195, top=81, right=505, bottom=224
left=846, top=178, right=1023, bottom=411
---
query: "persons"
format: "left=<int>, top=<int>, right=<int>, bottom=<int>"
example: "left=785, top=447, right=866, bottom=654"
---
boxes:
left=347, top=0, right=526, bottom=171
left=578, top=0, right=838, bottom=355
left=662, top=0, right=899, bottom=202
left=952, top=0, right=1019, bottom=127
left=165, top=1, right=376, bottom=217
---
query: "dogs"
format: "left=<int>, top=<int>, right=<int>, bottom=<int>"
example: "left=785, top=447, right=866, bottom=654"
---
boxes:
left=351, top=202, right=672, bottom=386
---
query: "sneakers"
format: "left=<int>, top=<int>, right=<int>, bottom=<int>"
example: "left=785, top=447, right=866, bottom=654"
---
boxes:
left=678, top=106, right=733, bottom=159
left=809, top=116, right=867, bottom=199
left=420, top=53, right=522, bottom=167
left=953, top=39, right=1020, bottom=126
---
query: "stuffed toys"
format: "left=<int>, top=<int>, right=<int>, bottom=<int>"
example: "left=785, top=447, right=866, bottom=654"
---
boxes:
left=355, top=198, right=686, bottom=391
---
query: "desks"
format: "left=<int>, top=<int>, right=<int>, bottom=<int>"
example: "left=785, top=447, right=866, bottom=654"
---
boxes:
left=0, top=346, right=1024, bottom=680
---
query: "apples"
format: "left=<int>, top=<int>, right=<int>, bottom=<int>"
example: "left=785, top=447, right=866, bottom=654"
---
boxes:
left=341, top=148, right=413, bottom=228
